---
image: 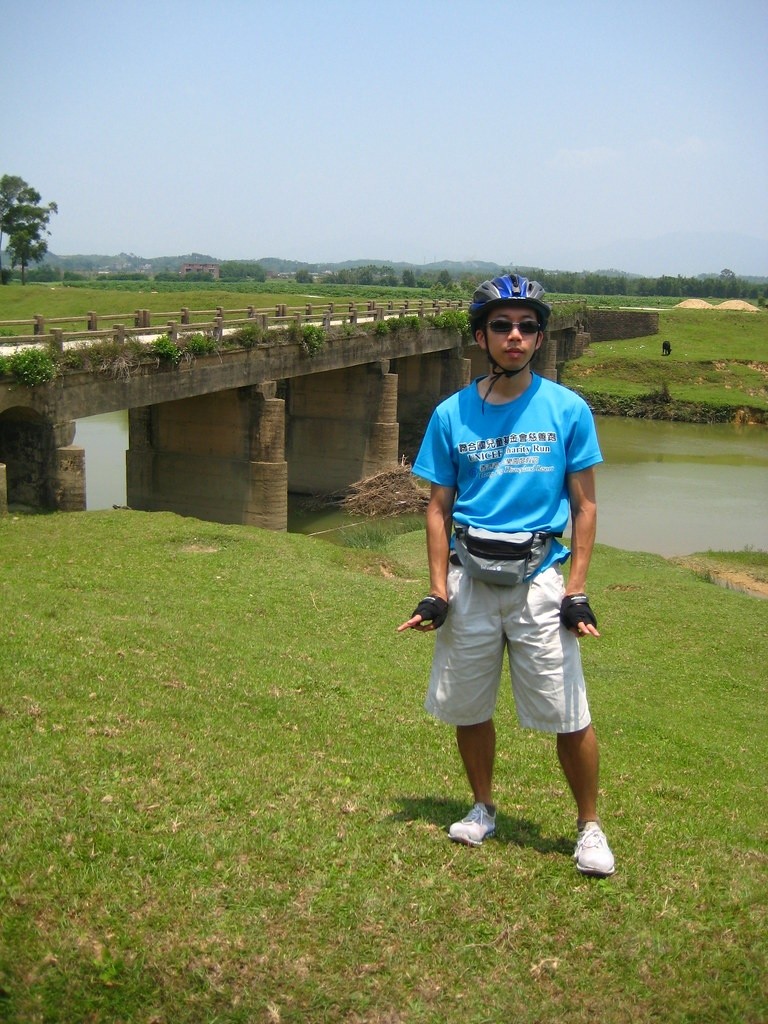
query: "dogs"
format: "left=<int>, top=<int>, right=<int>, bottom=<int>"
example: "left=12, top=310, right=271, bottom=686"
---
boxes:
left=663, top=341, right=672, bottom=356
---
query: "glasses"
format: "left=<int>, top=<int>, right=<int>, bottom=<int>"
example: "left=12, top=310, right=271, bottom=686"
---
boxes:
left=486, top=320, right=540, bottom=334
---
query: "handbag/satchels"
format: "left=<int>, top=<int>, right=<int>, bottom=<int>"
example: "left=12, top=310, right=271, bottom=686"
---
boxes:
left=453, top=520, right=562, bottom=586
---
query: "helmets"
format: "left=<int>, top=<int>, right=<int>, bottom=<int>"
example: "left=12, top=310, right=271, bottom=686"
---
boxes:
left=468, top=274, right=551, bottom=327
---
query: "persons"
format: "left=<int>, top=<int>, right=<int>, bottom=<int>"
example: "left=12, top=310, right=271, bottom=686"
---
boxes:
left=396, top=273, right=618, bottom=878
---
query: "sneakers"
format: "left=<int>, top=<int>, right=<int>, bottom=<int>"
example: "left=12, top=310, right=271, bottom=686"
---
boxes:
left=447, top=803, right=496, bottom=847
left=573, top=822, right=615, bottom=876
